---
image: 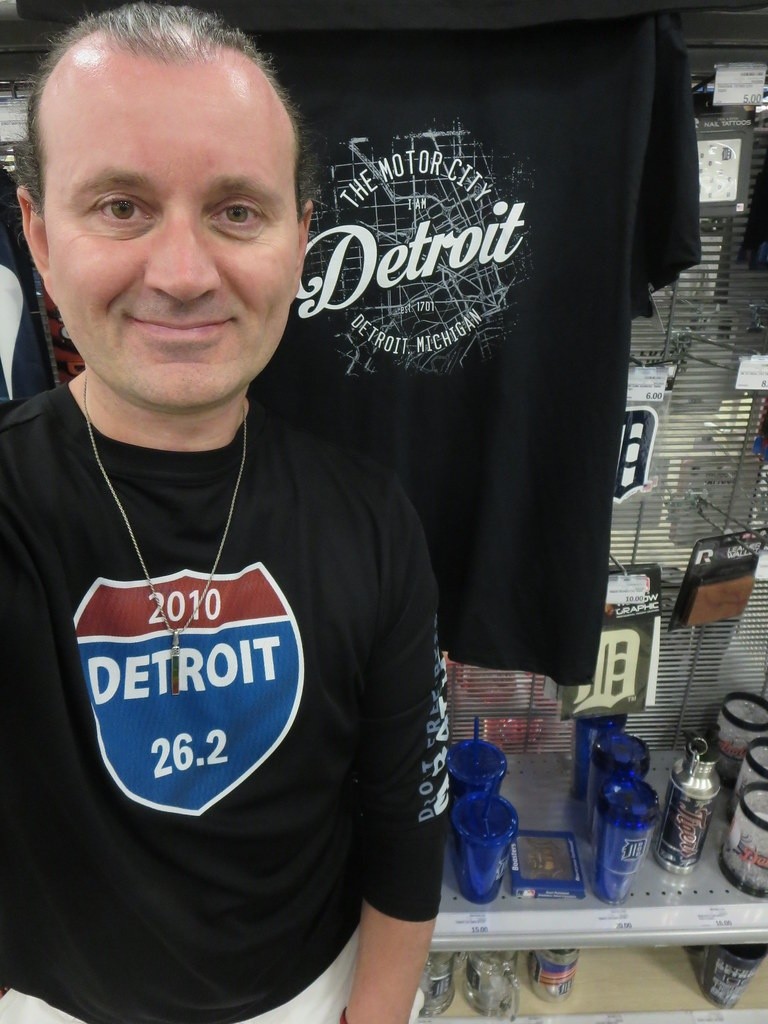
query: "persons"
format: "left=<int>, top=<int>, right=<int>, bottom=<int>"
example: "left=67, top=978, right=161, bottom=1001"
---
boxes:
left=0, top=0, right=449, bottom=1024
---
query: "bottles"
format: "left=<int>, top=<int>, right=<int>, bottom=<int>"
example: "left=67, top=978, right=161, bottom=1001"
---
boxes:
left=657, top=742, right=722, bottom=875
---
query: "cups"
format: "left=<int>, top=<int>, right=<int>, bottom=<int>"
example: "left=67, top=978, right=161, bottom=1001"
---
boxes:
left=419, top=951, right=521, bottom=1023
left=572, top=714, right=660, bottom=907
left=713, top=692, right=768, bottom=899
left=699, top=944, right=767, bottom=1009
left=445, top=739, right=517, bottom=903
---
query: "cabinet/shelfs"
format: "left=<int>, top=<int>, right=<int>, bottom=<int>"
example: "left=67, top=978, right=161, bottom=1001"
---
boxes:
left=414, top=747, right=768, bottom=1024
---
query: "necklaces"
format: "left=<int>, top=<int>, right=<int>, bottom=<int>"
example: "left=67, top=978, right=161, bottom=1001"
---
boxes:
left=82, top=370, right=248, bottom=695
